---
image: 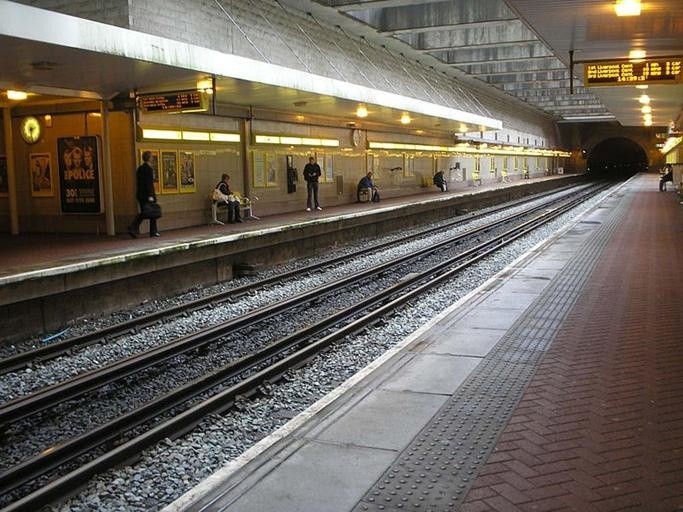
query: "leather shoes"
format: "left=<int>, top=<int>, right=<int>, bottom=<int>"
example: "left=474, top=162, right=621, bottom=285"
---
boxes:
left=227, top=219, right=244, bottom=224
left=128, top=226, right=139, bottom=239
left=150, top=232, right=160, bottom=238
left=305, top=206, right=323, bottom=212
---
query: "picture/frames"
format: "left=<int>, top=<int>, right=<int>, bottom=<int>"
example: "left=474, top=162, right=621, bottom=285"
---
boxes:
left=28, top=151, right=54, bottom=198
left=0, top=155, right=9, bottom=197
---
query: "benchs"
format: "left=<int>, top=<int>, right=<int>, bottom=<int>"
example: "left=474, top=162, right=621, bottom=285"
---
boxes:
left=206, top=192, right=261, bottom=225
left=356, top=183, right=379, bottom=203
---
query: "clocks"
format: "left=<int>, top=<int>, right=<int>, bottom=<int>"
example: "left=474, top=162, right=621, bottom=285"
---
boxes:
left=19, top=116, right=40, bottom=144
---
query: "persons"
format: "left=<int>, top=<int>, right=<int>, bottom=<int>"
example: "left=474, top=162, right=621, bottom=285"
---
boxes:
left=432, top=170, right=448, bottom=192
left=656, top=163, right=672, bottom=192
left=184, top=154, right=193, bottom=178
left=213, top=174, right=245, bottom=226
left=165, top=159, right=177, bottom=188
left=357, top=171, right=377, bottom=204
left=82, top=146, right=93, bottom=171
left=266, top=161, right=275, bottom=183
left=128, top=150, right=160, bottom=238
left=62, top=148, right=71, bottom=173
left=302, top=156, right=323, bottom=212
left=69, top=147, right=86, bottom=173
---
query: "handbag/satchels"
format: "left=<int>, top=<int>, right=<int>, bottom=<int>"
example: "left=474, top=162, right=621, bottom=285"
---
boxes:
left=212, top=183, right=227, bottom=201
left=373, top=192, right=379, bottom=201
left=142, top=202, right=161, bottom=219
